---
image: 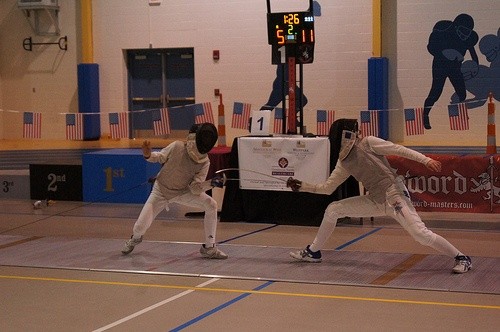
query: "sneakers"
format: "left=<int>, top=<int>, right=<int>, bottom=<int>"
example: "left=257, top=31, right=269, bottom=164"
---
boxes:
left=289, top=245, right=322, bottom=262
left=452, top=254, right=472, bottom=273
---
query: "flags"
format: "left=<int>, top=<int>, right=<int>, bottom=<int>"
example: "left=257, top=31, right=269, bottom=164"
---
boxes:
left=272, top=107, right=289, bottom=134
left=152, top=109, right=172, bottom=137
left=22, top=112, right=42, bottom=140
left=231, top=102, right=251, bottom=130
left=315, top=110, right=336, bottom=136
left=448, top=103, right=469, bottom=130
left=108, top=112, right=128, bottom=140
left=192, top=102, right=215, bottom=126
left=64, top=113, right=84, bottom=141
left=360, top=111, right=379, bottom=139
left=403, top=108, right=425, bottom=136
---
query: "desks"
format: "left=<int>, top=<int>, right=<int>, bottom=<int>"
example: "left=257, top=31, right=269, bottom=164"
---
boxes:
left=220, top=133, right=360, bottom=226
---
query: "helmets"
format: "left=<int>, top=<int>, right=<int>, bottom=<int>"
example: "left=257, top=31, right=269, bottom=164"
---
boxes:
left=194, top=123, right=218, bottom=154
left=329, top=118, right=359, bottom=162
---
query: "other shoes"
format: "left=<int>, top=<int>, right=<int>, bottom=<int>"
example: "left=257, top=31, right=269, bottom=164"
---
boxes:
left=120, top=234, right=143, bottom=256
left=200, top=243, right=228, bottom=259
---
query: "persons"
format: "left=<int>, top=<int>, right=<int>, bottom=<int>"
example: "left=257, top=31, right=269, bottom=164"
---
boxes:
left=290, top=118, right=472, bottom=274
left=120, top=122, right=228, bottom=260
